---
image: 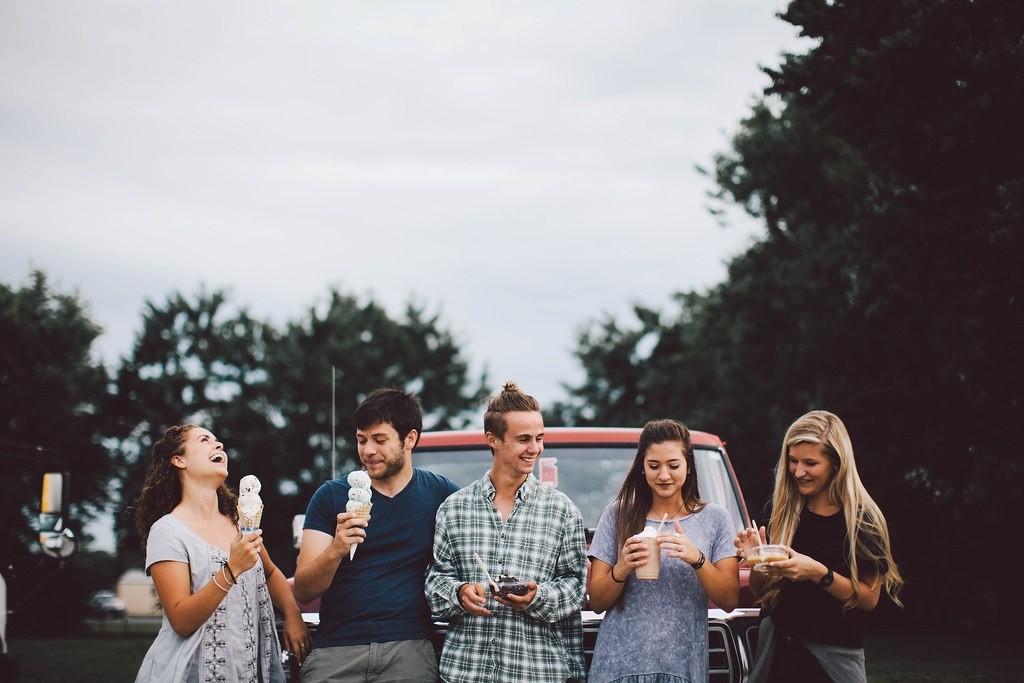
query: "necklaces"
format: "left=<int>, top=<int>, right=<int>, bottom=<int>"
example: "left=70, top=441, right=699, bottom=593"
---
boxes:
left=651, top=502, right=671, bottom=526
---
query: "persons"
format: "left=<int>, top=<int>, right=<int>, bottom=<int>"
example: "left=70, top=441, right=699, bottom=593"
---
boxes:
left=586, top=420, right=739, bottom=683
left=734, top=410, right=904, bottom=683
left=294, top=381, right=588, bottom=683
left=132, top=422, right=316, bottom=683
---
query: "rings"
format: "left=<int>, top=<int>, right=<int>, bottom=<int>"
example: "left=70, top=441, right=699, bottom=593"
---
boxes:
left=675, top=533, right=679, bottom=537
left=784, top=573, right=786, bottom=578
left=254, top=541, right=258, bottom=545
left=257, top=546, right=261, bottom=552
left=300, top=643, right=305, bottom=648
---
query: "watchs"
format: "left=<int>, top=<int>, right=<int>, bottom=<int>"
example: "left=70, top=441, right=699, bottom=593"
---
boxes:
left=817, top=567, right=834, bottom=589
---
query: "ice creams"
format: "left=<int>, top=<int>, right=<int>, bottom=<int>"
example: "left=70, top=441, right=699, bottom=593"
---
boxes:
left=632, top=526, right=660, bottom=539
left=489, top=573, right=528, bottom=598
left=346, top=469, right=373, bottom=560
left=743, top=544, right=790, bottom=566
left=236, top=475, right=263, bottom=540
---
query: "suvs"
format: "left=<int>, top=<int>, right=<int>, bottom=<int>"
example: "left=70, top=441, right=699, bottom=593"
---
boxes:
left=276, top=425, right=777, bottom=683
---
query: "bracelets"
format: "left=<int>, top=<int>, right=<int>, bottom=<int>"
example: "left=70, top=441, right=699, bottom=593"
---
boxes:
left=213, top=561, right=237, bottom=593
left=690, top=549, right=705, bottom=569
left=612, top=567, right=625, bottom=583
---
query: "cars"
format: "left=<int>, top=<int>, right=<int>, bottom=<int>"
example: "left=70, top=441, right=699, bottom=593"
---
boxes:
left=89, top=592, right=127, bottom=621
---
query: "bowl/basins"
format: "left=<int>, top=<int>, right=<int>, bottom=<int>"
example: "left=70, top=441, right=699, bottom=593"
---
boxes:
left=745, top=544, right=788, bottom=571
left=488, top=580, right=527, bottom=599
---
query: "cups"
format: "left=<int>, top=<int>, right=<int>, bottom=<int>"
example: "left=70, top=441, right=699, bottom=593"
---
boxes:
left=632, top=536, right=662, bottom=580
